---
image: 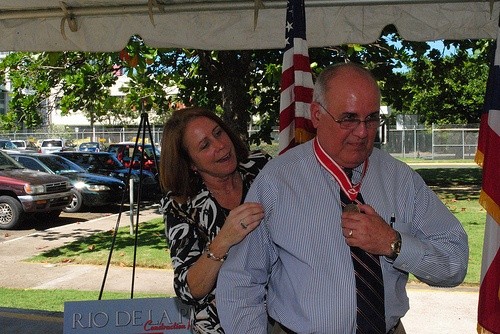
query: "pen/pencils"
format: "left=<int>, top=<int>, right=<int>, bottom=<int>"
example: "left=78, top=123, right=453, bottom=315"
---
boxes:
left=390, top=212, right=395, bottom=229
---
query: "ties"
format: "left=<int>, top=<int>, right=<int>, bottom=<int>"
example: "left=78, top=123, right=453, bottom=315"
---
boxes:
left=334, top=168, right=386, bottom=334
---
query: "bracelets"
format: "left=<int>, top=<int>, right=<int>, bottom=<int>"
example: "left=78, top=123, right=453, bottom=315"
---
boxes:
left=205, top=241, right=228, bottom=262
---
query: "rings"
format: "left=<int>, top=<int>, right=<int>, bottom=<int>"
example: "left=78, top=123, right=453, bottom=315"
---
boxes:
left=241, top=223, right=247, bottom=228
left=349, top=230, right=352, bottom=236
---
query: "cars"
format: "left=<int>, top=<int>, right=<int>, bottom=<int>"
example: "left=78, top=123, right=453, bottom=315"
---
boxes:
left=40, top=139, right=77, bottom=153
left=4, top=150, right=129, bottom=213
left=49, top=151, right=159, bottom=200
left=10, top=139, right=40, bottom=150
left=0, top=139, right=19, bottom=150
left=79, top=142, right=106, bottom=153
left=107, top=142, right=162, bottom=172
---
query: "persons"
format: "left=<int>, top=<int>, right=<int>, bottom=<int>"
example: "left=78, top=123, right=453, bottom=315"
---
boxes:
left=159, top=107, right=274, bottom=334
left=216, top=62, right=468, bottom=334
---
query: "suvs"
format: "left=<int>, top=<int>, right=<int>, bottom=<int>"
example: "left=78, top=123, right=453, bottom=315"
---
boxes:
left=0, top=150, right=73, bottom=230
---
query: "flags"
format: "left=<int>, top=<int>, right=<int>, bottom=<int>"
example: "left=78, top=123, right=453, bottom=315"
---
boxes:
left=278, top=0, right=315, bottom=155
left=474, top=36, right=500, bottom=334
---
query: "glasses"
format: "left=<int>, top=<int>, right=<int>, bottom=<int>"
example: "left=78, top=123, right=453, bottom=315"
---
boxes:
left=318, top=102, right=386, bottom=130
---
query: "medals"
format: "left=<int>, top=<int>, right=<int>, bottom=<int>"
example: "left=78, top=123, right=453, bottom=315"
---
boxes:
left=343, top=204, right=360, bottom=213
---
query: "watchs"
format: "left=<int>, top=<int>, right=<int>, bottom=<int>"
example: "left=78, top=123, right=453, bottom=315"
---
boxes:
left=390, top=232, right=402, bottom=260
